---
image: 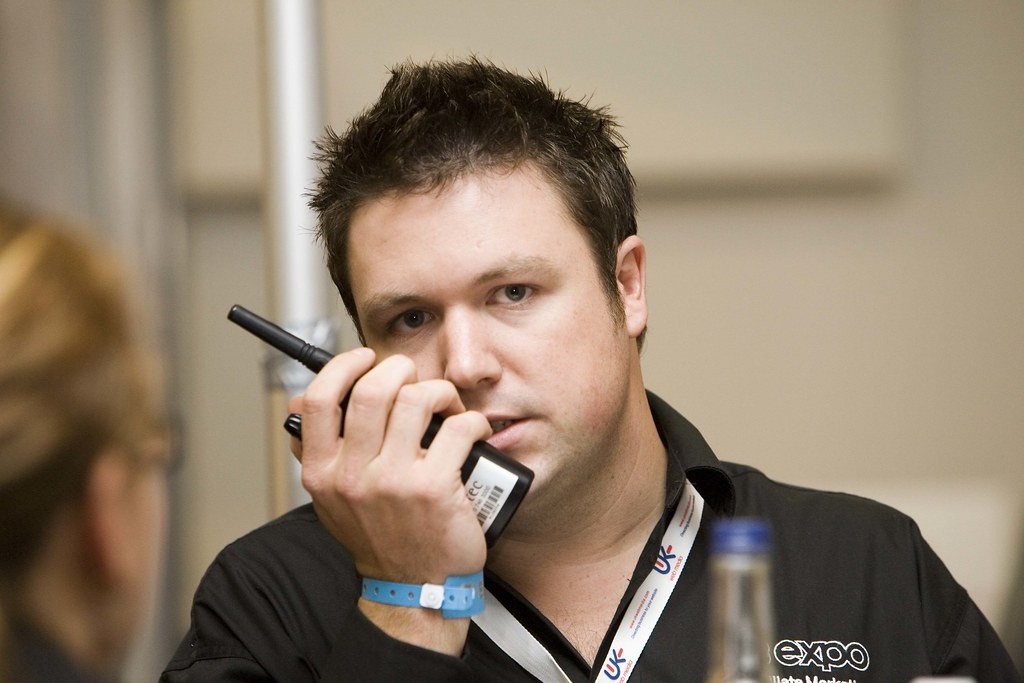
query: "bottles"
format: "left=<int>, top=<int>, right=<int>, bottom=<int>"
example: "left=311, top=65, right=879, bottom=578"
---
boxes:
left=705, top=517, right=780, bottom=683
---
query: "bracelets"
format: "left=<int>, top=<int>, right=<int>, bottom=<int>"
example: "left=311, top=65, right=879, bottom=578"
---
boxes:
left=361, top=570, right=485, bottom=620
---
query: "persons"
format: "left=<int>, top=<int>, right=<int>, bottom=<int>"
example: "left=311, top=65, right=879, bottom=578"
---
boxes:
left=156, top=55, right=1024, bottom=683
left=0, top=188, right=173, bottom=683
